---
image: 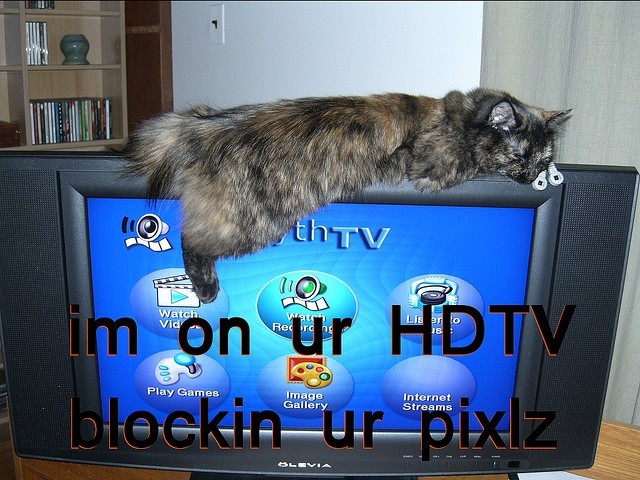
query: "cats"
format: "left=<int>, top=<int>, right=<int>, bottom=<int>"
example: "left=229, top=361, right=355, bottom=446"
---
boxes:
left=105, top=89, right=572, bottom=303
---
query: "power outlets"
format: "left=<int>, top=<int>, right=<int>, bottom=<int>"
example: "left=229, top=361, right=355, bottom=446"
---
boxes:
left=208, top=3, right=226, bottom=45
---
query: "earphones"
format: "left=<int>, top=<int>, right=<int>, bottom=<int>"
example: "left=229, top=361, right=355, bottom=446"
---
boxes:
left=533, top=163, right=564, bottom=194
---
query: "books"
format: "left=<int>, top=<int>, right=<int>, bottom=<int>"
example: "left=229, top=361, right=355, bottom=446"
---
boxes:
left=25, top=21, right=48, bottom=64
left=30, top=98, right=113, bottom=146
left=24, top=0, right=55, bottom=9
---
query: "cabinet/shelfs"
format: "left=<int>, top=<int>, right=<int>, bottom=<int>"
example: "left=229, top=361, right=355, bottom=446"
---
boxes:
left=0, top=0, right=129, bottom=152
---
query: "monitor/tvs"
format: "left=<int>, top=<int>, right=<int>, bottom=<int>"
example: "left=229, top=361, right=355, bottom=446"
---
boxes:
left=0, top=139, right=640, bottom=479
